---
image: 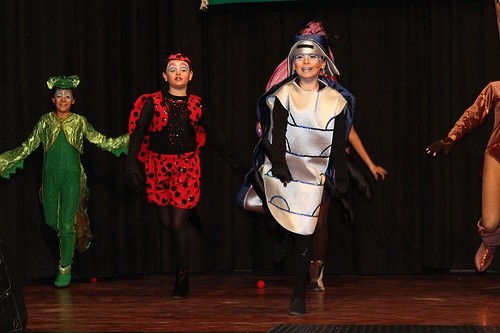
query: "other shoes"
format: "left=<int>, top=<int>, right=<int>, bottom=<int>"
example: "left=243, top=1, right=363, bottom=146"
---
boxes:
left=54, top=265, right=72, bottom=286
left=288, top=297, right=306, bottom=315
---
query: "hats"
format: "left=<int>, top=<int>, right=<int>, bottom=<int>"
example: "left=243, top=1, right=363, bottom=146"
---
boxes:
left=287, top=33, right=340, bottom=77
left=165, top=52, right=191, bottom=70
left=47, top=74, right=80, bottom=88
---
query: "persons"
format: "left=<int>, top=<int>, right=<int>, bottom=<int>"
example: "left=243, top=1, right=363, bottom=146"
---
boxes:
left=128, top=53, right=208, bottom=298
left=424, top=79, right=500, bottom=274
left=256, top=21, right=372, bottom=290
left=0, top=74, right=132, bottom=288
left=238, top=32, right=387, bottom=317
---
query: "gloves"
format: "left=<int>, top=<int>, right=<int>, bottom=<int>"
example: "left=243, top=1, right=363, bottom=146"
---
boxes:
left=271, top=160, right=292, bottom=182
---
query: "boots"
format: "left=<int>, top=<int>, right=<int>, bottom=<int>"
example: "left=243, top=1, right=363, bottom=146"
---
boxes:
left=170, top=264, right=190, bottom=298
left=474, top=218, right=500, bottom=272
left=310, top=259, right=325, bottom=290
